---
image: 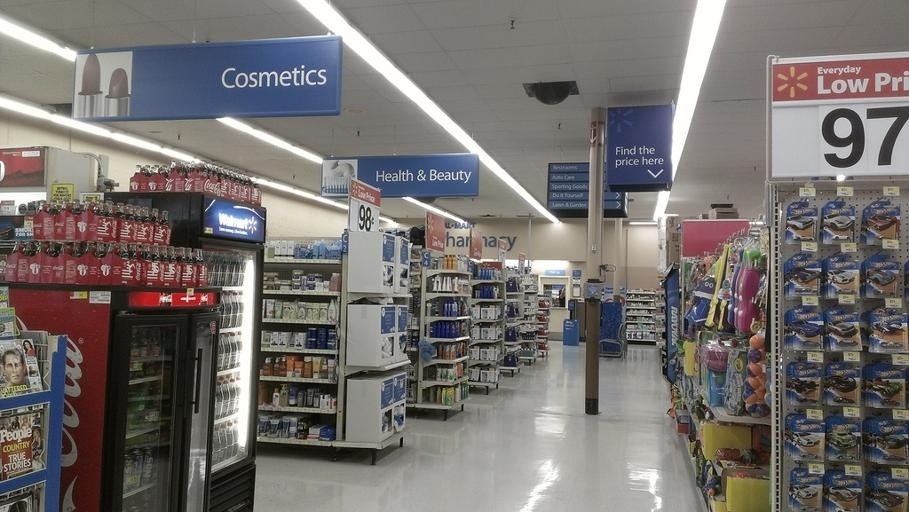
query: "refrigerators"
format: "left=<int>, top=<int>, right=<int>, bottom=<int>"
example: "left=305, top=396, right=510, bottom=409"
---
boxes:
left=0, top=282, right=223, bottom=512
left=104, top=192, right=267, bottom=512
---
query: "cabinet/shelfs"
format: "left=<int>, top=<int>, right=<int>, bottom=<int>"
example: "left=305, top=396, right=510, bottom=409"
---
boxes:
left=469, top=237, right=505, bottom=395
left=407, top=211, right=471, bottom=421
left=500, top=252, right=550, bottom=377
left=0, top=335, right=67, bottom=512
left=625, top=288, right=656, bottom=345
left=258, top=178, right=412, bottom=465
left=656, top=50, right=909, bottom=512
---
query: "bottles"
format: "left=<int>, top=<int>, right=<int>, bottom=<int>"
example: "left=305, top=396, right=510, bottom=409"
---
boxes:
left=3, top=201, right=208, bottom=290
left=207, top=248, right=245, bottom=465
left=133, top=160, right=262, bottom=206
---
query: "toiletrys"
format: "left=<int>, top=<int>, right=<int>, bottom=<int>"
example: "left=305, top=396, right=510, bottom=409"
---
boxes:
left=256, top=237, right=342, bottom=441
left=475, top=263, right=503, bottom=298
left=431, top=252, right=470, bottom=410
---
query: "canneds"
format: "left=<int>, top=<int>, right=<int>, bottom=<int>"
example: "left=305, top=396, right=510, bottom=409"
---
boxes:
left=122, top=446, right=154, bottom=495
